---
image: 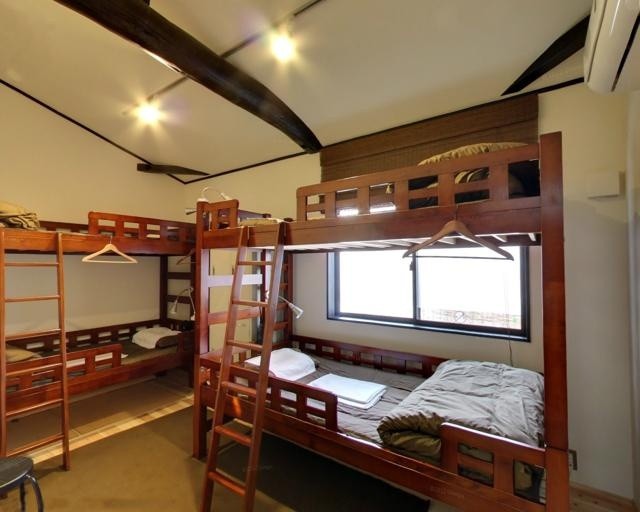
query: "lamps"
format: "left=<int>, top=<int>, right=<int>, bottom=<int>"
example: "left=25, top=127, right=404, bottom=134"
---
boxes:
left=196, top=186, right=233, bottom=203
left=170, top=287, right=196, bottom=322
left=278, top=295, right=304, bottom=319
left=184, top=208, right=196, bottom=215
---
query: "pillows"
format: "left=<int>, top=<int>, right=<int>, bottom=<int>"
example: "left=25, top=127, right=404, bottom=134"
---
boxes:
left=243, top=347, right=316, bottom=382
left=138, top=326, right=180, bottom=348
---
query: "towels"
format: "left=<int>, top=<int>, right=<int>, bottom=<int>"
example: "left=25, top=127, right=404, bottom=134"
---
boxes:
left=132, top=327, right=182, bottom=350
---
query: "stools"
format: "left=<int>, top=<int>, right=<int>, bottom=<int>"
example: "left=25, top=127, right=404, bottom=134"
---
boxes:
left=0, top=455, right=44, bottom=512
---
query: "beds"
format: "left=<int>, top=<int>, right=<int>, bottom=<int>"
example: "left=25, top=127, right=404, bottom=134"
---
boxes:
left=0, top=211, right=196, bottom=472
left=192, top=132, right=572, bottom=511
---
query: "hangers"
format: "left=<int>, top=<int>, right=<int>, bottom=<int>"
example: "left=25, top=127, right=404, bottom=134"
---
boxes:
left=402, top=205, right=514, bottom=260
left=176, top=240, right=196, bottom=265
left=81, top=236, right=138, bottom=264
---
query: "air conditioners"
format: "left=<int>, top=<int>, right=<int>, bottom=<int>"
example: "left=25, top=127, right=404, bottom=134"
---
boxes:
left=582, top=0, right=640, bottom=95
